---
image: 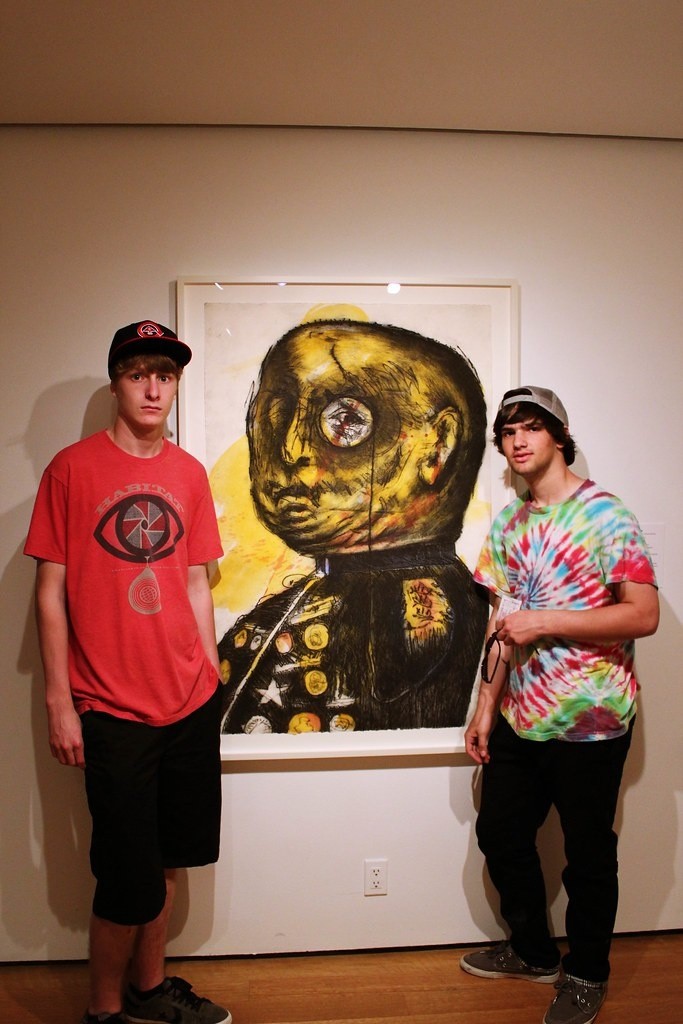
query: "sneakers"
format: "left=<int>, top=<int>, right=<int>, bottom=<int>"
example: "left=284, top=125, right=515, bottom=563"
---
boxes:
left=543, top=977, right=608, bottom=1024
left=123, top=976, right=232, bottom=1024
left=82, top=1010, right=131, bottom=1024
left=461, top=943, right=561, bottom=985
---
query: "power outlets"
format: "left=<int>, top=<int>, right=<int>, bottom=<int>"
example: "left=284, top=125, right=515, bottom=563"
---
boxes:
left=364, top=859, right=387, bottom=896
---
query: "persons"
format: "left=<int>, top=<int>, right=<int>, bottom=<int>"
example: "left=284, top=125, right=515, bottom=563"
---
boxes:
left=461, top=385, right=660, bottom=1024
left=23, top=320, right=233, bottom=1024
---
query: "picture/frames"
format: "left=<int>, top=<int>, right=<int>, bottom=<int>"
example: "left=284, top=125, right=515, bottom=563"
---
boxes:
left=173, top=276, right=521, bottom=762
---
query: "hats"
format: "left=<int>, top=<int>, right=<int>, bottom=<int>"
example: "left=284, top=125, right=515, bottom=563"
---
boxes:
left=499, top=386, right=576, bottom=466
left=108, top=320, right=192, bottom=380
